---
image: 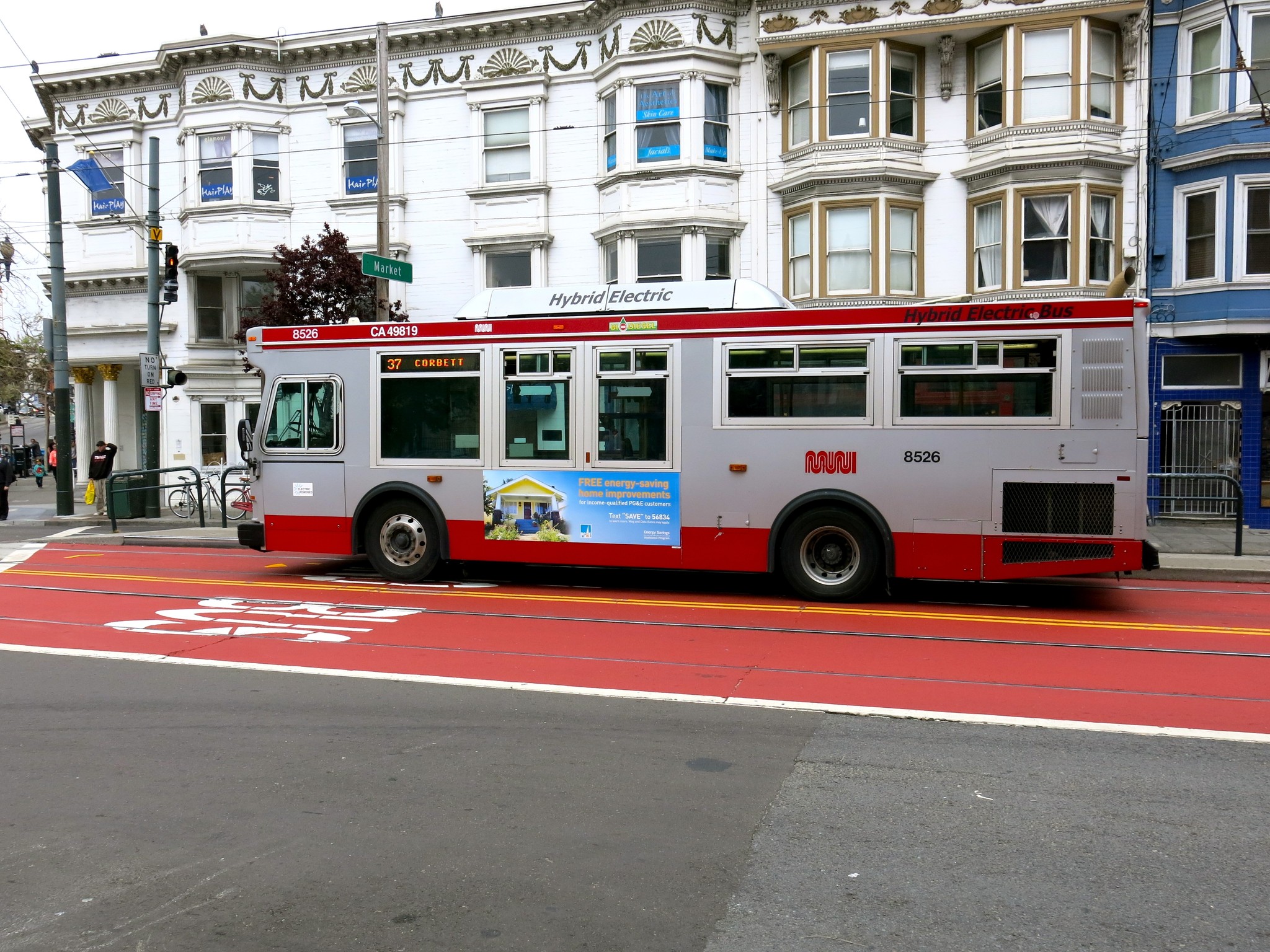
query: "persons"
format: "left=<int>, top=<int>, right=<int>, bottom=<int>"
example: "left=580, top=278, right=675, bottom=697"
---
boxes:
left=0, top=449, right=13, bottom=520
left=49, top=444, right=57, bottom=483
left=24, top=439, right=43, bottom=462
left=88, top=440, right=117, bottom=516
left=47, top=436, right=56, bottom=472
left=71, top=440, right=77, bottom=477
left=620, top=438, right=634, bottom=457
left=604, top=429, right=618, bottom=450
left=33, top=459, right=48, bottom=488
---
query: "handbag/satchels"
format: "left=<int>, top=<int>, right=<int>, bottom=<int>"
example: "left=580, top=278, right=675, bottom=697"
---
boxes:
left=84, top=480, right=95, bottom=505
left=10, top=471, right=16, bottom=483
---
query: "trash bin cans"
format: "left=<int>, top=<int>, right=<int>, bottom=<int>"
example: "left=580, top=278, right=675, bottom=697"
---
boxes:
left=14, top=447, right=32, bottom=478
left=105, top=469, right=146, bottom=519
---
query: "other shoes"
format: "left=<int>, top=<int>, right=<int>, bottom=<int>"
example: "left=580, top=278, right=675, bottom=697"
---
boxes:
left=103, top=512, right=107, bottom=516
left=0, top=517, right=6, bottom=521
left=93, top=513, right=99, bottom=516
left=22, top=476, right=26, bottom=478
left=18, top=477, right=22, bottom=478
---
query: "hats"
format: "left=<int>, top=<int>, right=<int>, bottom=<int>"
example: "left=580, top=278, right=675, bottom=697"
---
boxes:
left=36, top=459, right=41, bottom=462
left=96, top=440, right=105, bottom=446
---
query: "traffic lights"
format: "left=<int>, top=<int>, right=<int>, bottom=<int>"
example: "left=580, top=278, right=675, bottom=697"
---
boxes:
left=168, top=370, right=189, bottom=386
left=163, top=292, right=178, bottom=302
left=164, top=244, right=179, bottom=290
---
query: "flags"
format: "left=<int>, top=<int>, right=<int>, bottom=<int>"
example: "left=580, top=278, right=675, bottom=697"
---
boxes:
left=65, top=159, right=114, bottom=193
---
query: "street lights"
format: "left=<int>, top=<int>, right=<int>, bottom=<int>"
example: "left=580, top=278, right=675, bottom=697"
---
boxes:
left=344, top=21, right=395, bottom=320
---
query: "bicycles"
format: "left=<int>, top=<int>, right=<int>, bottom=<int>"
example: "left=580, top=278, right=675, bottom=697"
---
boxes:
left=168, top=473, right=222, bottom=518
left=222, top=477, right=254, bottom=520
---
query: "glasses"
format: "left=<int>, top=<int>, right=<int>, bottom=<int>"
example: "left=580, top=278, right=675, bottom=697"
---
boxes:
left=97, top=445, right=103, bottom=447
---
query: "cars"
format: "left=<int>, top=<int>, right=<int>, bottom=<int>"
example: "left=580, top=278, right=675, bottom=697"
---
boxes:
left=31, top=449, right=45, bottom=469
left=1, top=447, right=31, bottom=477
left=0, top=402, right=45, bottom=417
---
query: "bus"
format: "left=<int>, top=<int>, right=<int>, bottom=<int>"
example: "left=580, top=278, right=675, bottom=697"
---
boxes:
left=236, top=264, right=1155, bottom=604
